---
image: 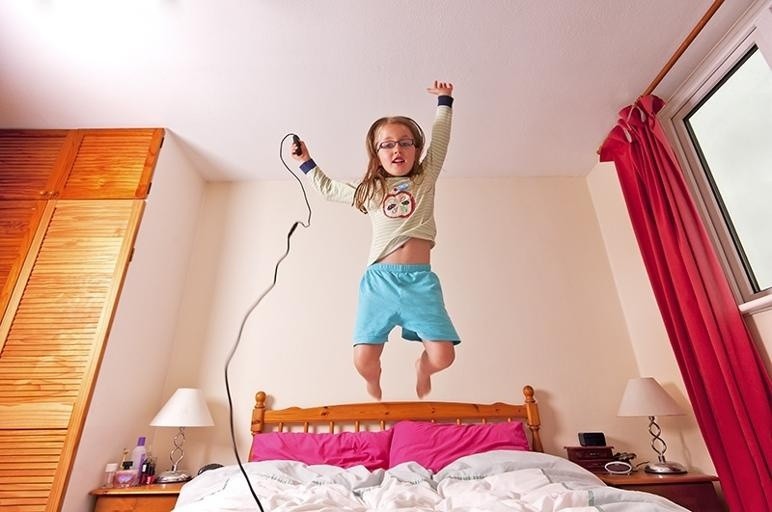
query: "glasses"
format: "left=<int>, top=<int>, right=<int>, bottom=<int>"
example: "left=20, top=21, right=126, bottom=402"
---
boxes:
left=375, top=139, right=414, bottom=152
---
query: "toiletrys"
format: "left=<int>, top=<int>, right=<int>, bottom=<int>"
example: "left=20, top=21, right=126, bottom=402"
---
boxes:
left=103, top=436, right=157, bottom=488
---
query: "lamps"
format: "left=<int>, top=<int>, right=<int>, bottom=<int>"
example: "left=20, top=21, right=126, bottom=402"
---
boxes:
left=616, top=377, right=687, bottom=474
left=149, top=388, right=215, bottom=482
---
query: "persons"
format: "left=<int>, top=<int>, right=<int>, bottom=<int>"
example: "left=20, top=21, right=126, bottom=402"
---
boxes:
left=290, top=78, right=462, bottom=400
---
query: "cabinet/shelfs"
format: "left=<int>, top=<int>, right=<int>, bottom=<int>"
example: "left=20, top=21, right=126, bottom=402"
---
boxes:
left=0, top=128, right=204, bottom=512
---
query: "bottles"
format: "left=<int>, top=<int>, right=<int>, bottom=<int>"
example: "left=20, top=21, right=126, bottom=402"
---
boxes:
left=104, top=462, right=117, bottom=488
left=130, top=436, right=146, bottom=483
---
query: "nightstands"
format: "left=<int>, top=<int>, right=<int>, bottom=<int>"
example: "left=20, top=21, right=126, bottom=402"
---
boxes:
left=88, top=476, right=188, bottom=512
left=594, top=465, right=720, bottom=511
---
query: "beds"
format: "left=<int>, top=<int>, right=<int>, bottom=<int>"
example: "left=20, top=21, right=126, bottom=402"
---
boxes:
left=174, top=385, right=634, bottom=512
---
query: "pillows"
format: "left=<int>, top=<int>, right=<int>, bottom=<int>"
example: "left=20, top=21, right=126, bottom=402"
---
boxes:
left=248, top=427, right=393, bottom=469
left=388, top=420, right=528, bottom=474
